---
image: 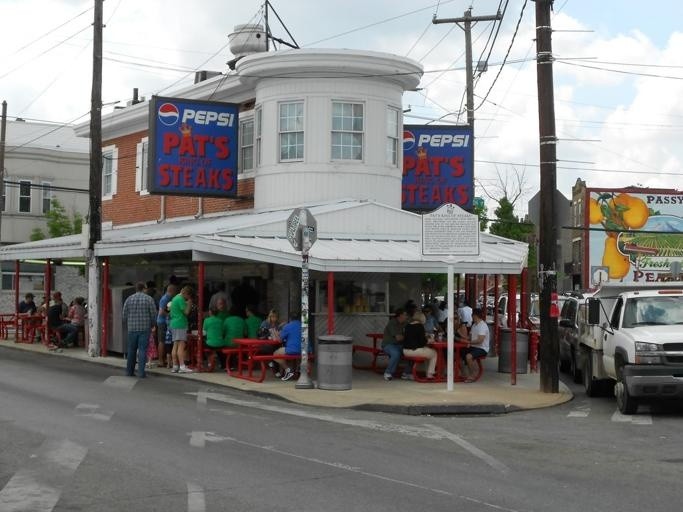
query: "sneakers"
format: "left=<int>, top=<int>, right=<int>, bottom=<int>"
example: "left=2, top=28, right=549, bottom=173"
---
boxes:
left=383, top=370, right=439, bottom=381
left=281, top=368, right=294, bottom=381
left=156, top=361, right=194, bottom=373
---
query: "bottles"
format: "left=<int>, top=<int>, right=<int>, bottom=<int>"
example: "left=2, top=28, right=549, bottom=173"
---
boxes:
left=425, top=330, right=442, bottom=342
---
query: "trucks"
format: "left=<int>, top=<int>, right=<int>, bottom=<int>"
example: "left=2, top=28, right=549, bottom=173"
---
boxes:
left=575, top=279, right=682, bottom=417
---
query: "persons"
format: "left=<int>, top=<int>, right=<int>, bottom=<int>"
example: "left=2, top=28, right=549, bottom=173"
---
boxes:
left=17, top=290, right=88, bottom=350
left=493, top=301, right=503, bottom=326
left=380, top=297, right=490, bottom=383
left=517, top=312, right=528, bottom=334
left=121, top=275, right=313, bottom=381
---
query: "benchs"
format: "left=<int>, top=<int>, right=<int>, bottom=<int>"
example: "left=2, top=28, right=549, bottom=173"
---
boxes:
left=189, top=336, right=314, bottom=382
left=353, top=334, right=483, bottom=384
left=2, top=311, right=71, bottom=348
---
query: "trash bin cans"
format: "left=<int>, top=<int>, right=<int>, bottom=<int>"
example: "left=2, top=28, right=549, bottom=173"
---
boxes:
left=317, top=335, right=353, bottom=390
left=498, top=328, right=529, bottom=374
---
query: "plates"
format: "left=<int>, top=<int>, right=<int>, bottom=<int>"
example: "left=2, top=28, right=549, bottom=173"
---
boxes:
left=486, top=320, right=495, bottom=324
left=257, top=335, right=272, bottom=340
left=191, top=330, right=204, bottom=335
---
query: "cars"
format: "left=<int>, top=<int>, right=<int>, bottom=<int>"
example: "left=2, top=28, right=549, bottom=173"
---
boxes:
left=436, top=288, right=595, bottom=386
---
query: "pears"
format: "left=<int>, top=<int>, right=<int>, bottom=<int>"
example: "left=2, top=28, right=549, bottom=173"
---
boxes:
left=590, top=193, right=649, bottom=237
left=602, top=237, right=630, bottom=279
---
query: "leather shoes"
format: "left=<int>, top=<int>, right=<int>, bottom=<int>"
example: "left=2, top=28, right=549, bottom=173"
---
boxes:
left=126, top=372, right=148, bottom=378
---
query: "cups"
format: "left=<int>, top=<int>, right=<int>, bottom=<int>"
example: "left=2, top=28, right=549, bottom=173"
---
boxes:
left=28, top=310, right=32, bottom=315
